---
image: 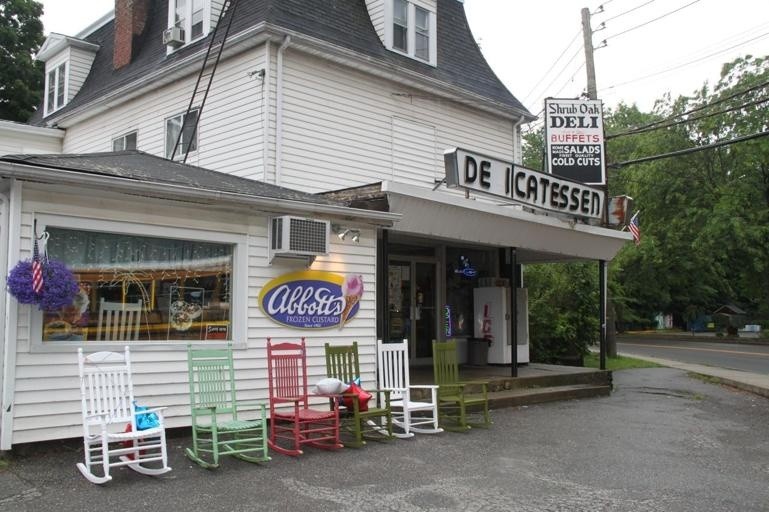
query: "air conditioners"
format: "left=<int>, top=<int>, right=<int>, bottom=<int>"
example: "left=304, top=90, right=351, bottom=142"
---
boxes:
left=272, top=215, right=329, bottom=257
left=163, top=27, right=186, bottom=49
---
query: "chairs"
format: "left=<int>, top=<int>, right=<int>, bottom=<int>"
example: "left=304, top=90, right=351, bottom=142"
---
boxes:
left=96, top=297, right=143, bottom=341
left=185, top=343, right=272, bottom=469
left=325, top=340, right=396, bottom=449
left=76, top=346, right=172, bottom=485
left=265, top=336, right=343, bottom=457
left=365, top=338, right=443, bottom=439
left=425, top=339, right=491, bottom=433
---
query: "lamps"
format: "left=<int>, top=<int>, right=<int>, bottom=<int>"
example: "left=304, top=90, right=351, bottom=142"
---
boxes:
left=333, top=225, right=362, bottom=243
